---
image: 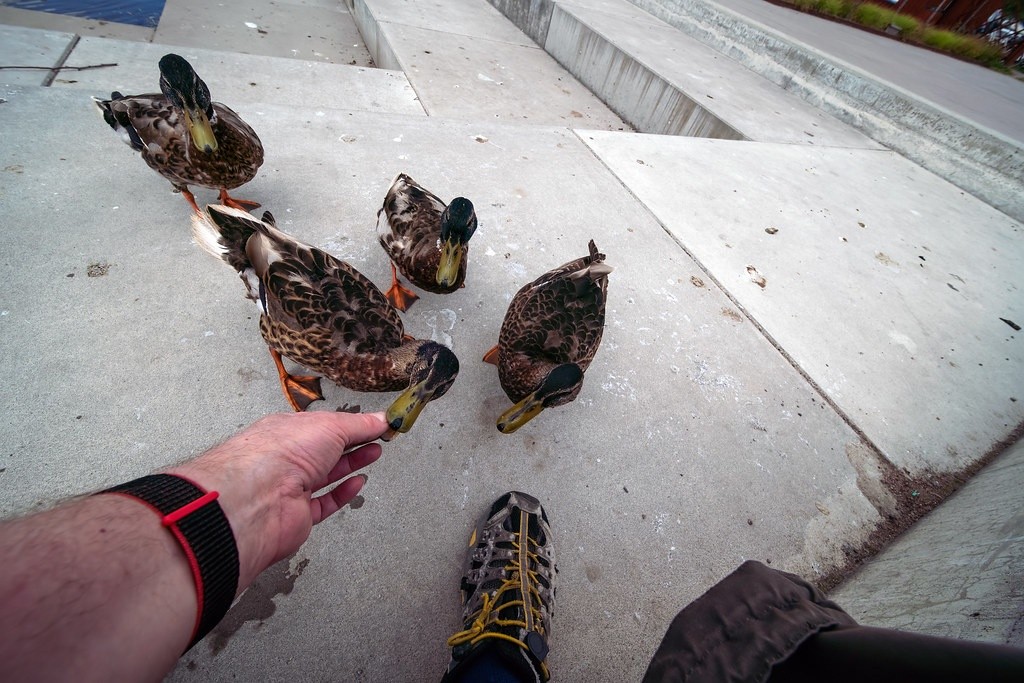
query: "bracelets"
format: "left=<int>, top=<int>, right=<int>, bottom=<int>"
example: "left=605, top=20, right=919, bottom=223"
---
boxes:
left=89, top=473, right=241, bottom=659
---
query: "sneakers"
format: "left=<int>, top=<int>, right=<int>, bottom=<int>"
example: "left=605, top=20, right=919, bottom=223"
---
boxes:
left=448, top=491, right=559, bottom=683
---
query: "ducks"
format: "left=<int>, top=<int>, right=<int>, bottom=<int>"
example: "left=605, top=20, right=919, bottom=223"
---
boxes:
left=375, top=171, right=478, bottom=314
left=187, top=200, right=461, bottom=441
left=91, top=52, right=265, bottom=220
left=482, top=239, right=614, bottom=434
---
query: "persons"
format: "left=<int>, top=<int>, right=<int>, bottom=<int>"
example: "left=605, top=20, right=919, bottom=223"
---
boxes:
left=0, top=411, right=559, bottom=683
